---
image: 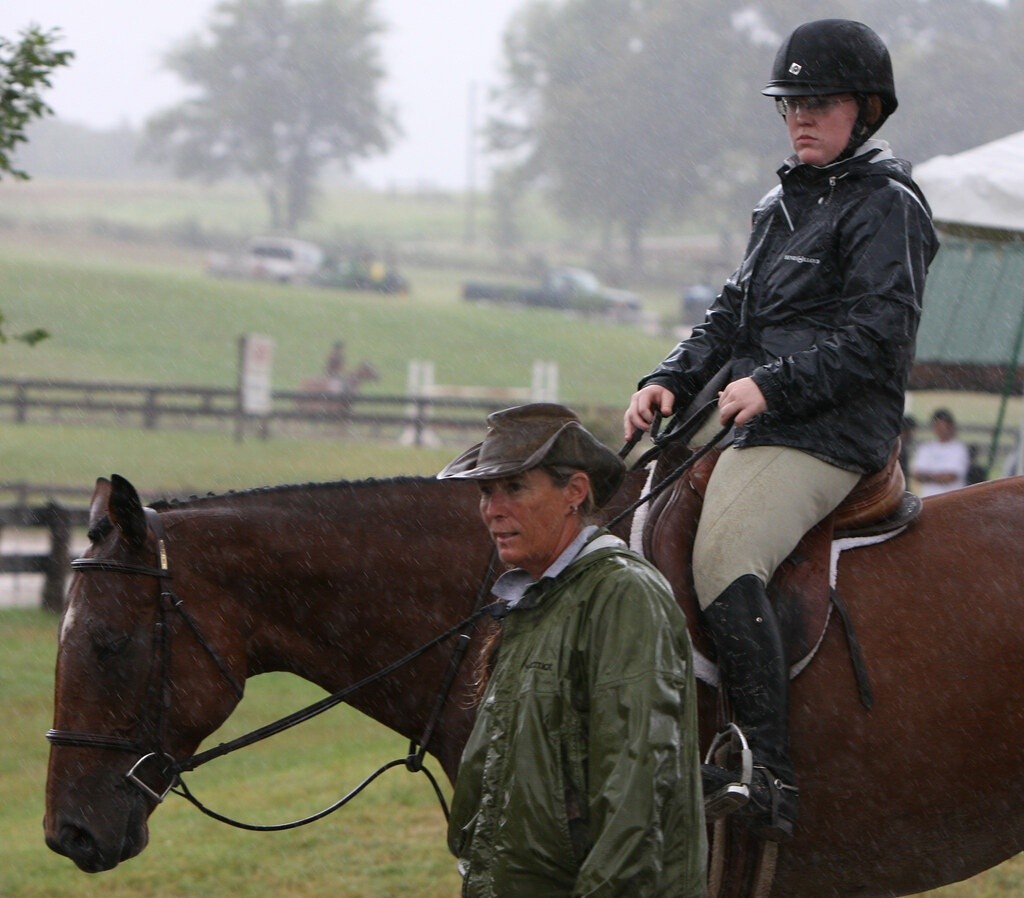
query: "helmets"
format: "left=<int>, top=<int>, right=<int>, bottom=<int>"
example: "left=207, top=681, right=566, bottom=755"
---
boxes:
left=761, top=18, right=899, bottom=116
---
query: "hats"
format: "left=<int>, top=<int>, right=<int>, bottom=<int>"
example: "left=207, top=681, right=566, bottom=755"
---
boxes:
left=436, top=403, right=628, bottom=513
left=932, top=409, right=954, bottom=422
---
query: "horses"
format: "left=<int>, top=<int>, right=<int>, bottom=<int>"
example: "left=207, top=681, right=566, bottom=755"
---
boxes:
left=297, top=361, right=382, bottom=433
left=42, top=475, right=1024, bottom=897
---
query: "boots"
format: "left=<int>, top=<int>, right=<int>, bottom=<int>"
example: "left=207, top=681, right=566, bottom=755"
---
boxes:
left=697, top=574, right=803, bottom=819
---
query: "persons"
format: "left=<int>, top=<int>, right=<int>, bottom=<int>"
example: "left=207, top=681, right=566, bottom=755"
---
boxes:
left=434, top=401, right=708, bottom=898
left=900, top=407, right=987, bottom=497
left=623, top=17, right=939, bottom=839
left=326, top=341, right=346, bottom=391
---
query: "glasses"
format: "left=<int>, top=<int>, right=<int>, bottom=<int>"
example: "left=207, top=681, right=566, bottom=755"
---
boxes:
left=776, top=97, right=856, bottom=115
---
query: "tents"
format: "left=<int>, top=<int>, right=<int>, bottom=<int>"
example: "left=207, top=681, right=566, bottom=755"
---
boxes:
left=912, top=132, right=1024, bottom=478
